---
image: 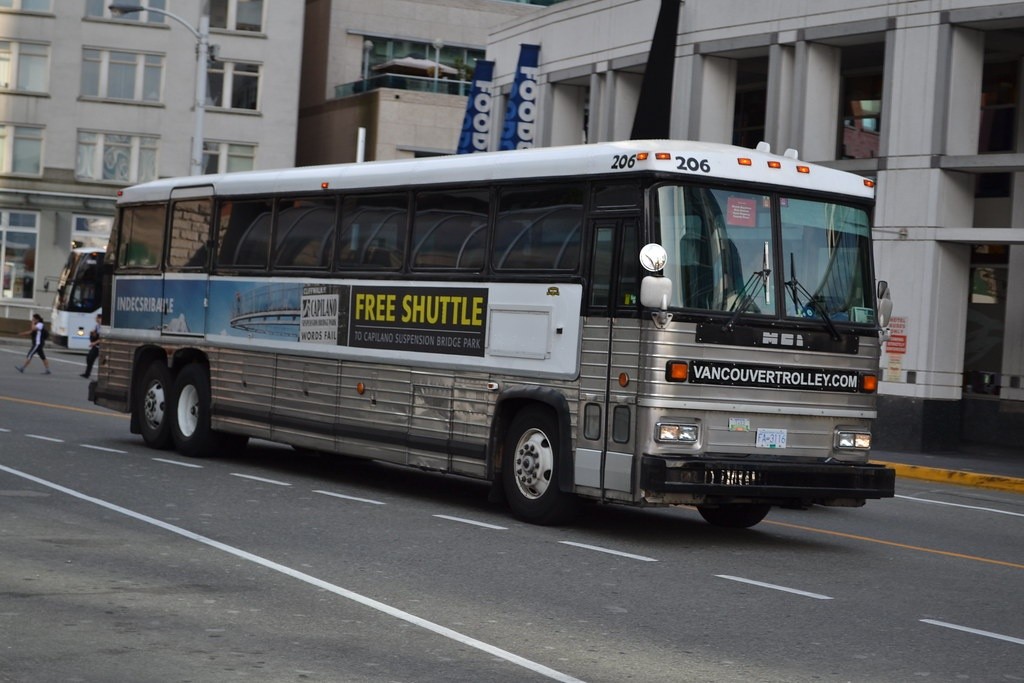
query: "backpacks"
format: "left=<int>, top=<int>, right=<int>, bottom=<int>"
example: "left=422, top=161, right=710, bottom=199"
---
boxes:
left=35, top=321, right=49, bottom=339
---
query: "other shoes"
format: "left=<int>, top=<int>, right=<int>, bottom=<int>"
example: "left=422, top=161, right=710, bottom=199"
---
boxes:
left=41, top=369, right=51, bottom=374
left=15, top=365, right=23, bottom=373
left=80, top=374, right=89, bottom=379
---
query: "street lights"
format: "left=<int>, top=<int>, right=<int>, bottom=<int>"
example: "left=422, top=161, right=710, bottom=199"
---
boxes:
left=108, top=0, right=211, bottom=175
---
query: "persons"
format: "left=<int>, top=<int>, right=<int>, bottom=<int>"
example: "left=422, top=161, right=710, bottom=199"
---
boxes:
left=14, top=313, right=51, bottom=375
left=80, top=314, right=102, bottom=378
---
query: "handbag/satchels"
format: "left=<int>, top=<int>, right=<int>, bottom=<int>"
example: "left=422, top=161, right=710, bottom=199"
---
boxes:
left=90, top=325, right=99, bottom=346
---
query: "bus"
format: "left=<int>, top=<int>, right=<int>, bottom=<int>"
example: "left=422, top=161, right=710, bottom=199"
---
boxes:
left=48, top=240, right=109, bottom=352
left=88, top=139, right=896, bottom=528
